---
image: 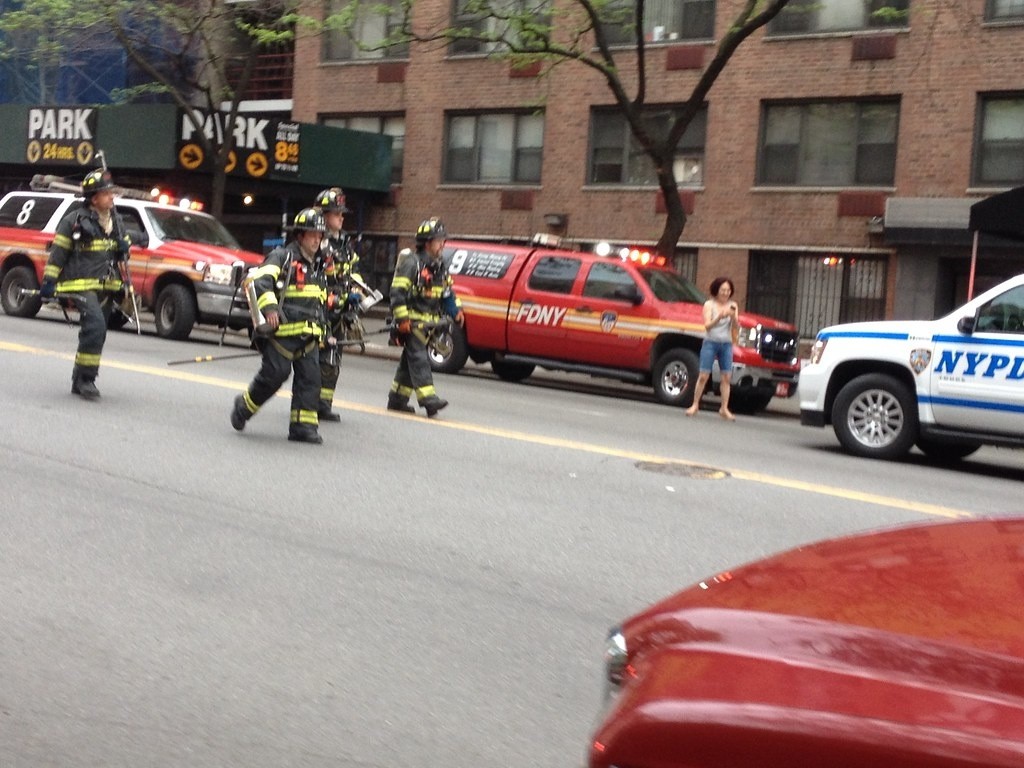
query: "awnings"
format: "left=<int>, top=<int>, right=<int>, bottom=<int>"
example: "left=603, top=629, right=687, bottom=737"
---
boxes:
left=884, top=196, right=1022, bottom=247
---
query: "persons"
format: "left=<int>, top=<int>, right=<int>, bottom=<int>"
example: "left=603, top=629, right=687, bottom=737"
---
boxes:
left=315, top=188, right=359, bottom=421
left=231, top=209, right=326, bottom=444
left=387, top=217, right=466, bottom=418
left=40, top=169, right=132, bottom=397
left=684, top=276, right=738, bottom=421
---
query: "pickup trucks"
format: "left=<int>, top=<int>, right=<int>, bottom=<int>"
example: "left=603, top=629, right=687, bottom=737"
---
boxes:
left=414, top=231, right=802, bottom=415
left=1, top=175, right=264, bottom=337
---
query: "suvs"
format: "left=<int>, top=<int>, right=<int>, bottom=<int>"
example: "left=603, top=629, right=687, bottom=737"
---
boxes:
left=799, top=270, right=1024, bottom=476
left=592, top=514, right=1023, bottom=768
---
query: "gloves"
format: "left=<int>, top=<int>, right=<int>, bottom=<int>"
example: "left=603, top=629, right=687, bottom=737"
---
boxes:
left=454, top=310, right=465, bottom=328
left=116, top=236, right=129, bottom=253
left=39, top=283, right=55, bottom=305
left=399, top=319, right=412, bottom=334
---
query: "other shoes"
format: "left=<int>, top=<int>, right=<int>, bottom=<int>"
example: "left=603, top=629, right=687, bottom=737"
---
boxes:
left=387, top=401, right=416, bottom=412
left=287, top=429, right=324, bottom=443
left=231, top=394, right=246, bottom=431
left=426, top=399, right=448, bottom=418
left=71, top=376, right=100, bottom=398
left=319, top=409, right=341, bottom=422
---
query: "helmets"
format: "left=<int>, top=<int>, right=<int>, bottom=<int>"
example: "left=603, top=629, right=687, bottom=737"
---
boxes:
left=292, top=208, right=328, bottom=232
left=81, top=166, right=116, bottom=193
left=313, top=189, right=349, bottom=212
left=414, top=217, right=446, bottom=238
